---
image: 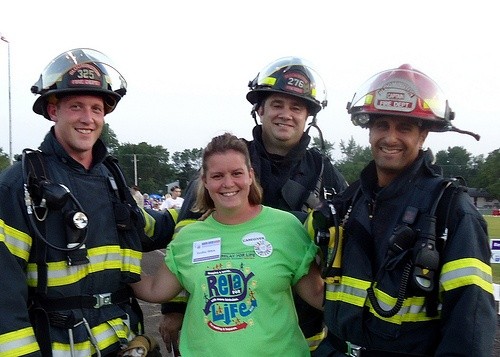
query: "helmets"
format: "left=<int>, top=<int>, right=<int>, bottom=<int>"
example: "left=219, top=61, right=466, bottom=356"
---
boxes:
left=32, top=51, right=121, bottom=122
left=358, top=64, right=445, bottom=133
left=246, top=60, right=322, bottom=116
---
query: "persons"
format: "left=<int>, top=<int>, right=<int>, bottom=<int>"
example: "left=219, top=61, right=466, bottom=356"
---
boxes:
left=0, top=46, right=185, bottom=357
left=127, top=183, right=184, bottom=214
left=135, top=131, right=326, bottom=357
left=302, top=63, right=499, bottom=357
left=157, top=54, right=349, bottom=353
